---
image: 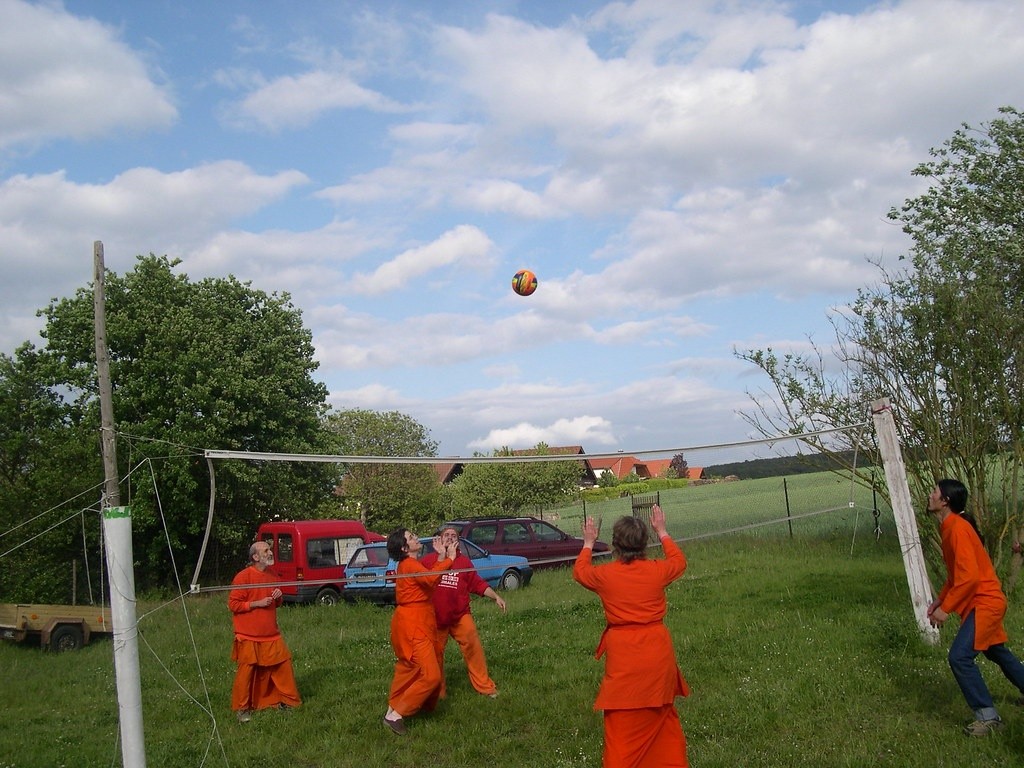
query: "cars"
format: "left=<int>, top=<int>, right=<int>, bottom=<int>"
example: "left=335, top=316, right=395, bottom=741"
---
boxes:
left=342, top=534, right=534, bottom=607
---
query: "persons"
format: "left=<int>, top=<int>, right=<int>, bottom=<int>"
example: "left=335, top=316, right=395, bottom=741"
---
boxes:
left=228, top=541, right=303, bottom=722
left=927, top=479, right=1024, bottom=735
left=383, top=527, right=507, bottom=735
left=572, top=503, right=687, bottom=768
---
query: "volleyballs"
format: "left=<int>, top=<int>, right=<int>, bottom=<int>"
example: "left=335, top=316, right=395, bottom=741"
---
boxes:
left=511, top=270, right=538, bottom=297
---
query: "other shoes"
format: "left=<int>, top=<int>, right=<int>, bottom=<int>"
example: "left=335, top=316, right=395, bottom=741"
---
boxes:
left=271, top=704, right=284, bottom=711
left=384, top=715, right=408, bottom=737
left=488, top=689, right=499, bottom=699
left=237, top=710, right=253, bottom=721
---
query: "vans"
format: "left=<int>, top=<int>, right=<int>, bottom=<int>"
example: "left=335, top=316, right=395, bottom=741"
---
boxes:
left=257, top=519, right=389, bottom=607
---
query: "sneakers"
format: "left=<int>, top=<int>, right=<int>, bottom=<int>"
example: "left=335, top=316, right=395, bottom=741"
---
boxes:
left=964, top=715, right=1002, bottom=737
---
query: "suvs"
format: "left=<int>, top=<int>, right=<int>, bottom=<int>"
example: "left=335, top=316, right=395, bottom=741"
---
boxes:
left=433, top=515, right=613, bottom=571
